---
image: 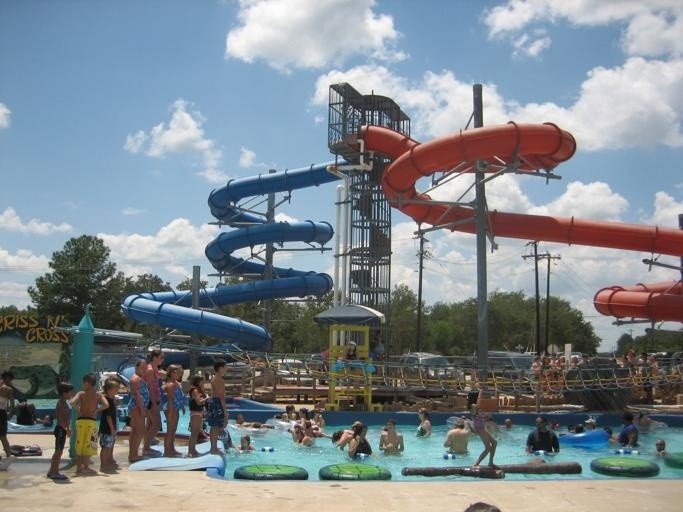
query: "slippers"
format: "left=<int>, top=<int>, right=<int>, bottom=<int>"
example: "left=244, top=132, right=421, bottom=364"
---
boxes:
left=47, top=471, right=68, bottom=480
left=7, top=454, right=14, bottom=465
